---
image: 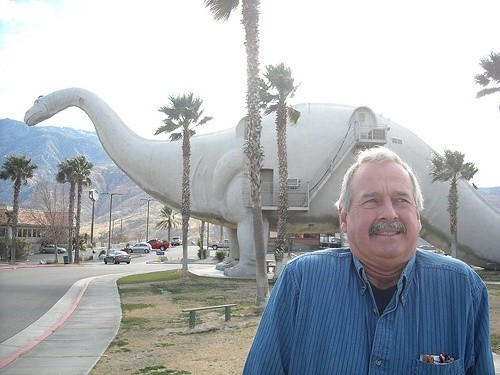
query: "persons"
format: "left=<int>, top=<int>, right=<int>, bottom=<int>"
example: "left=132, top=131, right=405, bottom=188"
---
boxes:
left=241, top=146, right=496, bottom=375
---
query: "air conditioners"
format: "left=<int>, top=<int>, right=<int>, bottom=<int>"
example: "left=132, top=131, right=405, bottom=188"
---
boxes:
left=286, top=177, right=299, bottom=187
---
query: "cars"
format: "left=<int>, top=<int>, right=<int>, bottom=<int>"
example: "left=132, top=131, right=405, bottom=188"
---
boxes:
left=40, top=245, right=66, bottom=254
left=103, top=251, right=131, bottom=264
left=120, top=242, right=152, bottom=254
left=147, top=238, right=170, bottom=251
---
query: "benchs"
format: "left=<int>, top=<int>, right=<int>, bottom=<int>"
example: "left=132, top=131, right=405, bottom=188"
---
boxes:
left=266, top=265, right=276, bottom=273
left=182, top=304, right=237, bottom=327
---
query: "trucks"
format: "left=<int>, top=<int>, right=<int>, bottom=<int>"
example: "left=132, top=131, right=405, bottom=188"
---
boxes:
left=171, top=237, right=182, bottom=246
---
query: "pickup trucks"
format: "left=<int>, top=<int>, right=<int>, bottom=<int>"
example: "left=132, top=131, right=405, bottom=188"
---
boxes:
left=209, top=239, right=229, bottom=250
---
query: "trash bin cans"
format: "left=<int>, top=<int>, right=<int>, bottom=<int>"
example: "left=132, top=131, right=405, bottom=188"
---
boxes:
left=63, top=256, right=68, bottom=264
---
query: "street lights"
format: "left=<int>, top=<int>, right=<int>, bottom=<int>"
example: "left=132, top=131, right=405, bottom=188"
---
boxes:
left=139, top=198, right=155, bottom=242
left=100, top=192, right=123, bottom=254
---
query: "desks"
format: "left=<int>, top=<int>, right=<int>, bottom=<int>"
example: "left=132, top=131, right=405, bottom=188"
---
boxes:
left=266, top=261, right=275, bottom=273
